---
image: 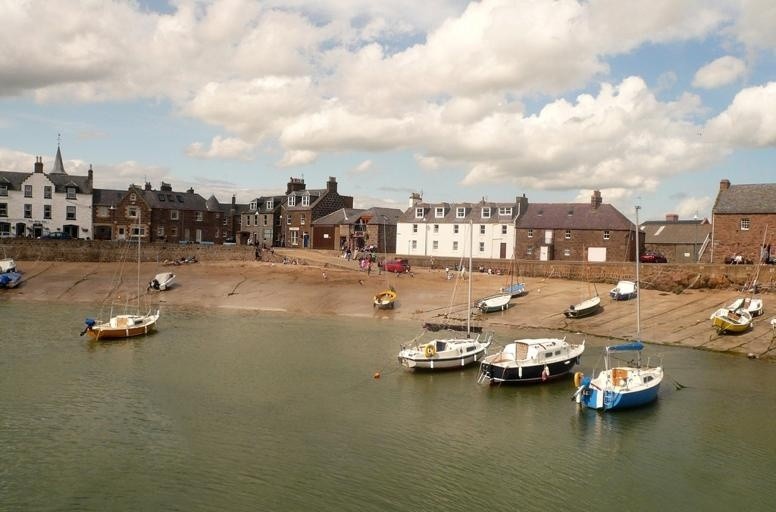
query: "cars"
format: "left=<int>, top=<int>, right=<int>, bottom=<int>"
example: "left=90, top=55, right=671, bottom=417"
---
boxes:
left=384, top=258, right=410, bottom=273
left=38, top=232, right=73, bottom=240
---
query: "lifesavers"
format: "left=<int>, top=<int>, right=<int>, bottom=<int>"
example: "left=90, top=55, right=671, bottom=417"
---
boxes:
left=424, top=344, right=436, bottom=358
left=573, top=372, right=584, bottom=389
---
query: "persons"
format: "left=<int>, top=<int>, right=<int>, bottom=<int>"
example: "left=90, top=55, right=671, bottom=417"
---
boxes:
left=733, top=253, right=743, bottom=265
left=343, top=244, right=384, bottom=276
left=760, top=244, right=765, bottom=265
left=445, top=265, right=449, bottom=274
left=762, top=245, right=768, bottom=266
left=729, top=252, right=736, bottom=264
left=488, top=267, right=492, bottom=277
left=245, top=235, right=275, bottom=260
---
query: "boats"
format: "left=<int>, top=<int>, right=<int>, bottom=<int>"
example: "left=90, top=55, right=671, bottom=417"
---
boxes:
left=479, top=339, right=586, bottom=384
left=374, top=289, right=397, bottom=308
left=0, top=258, right=22, bottom=289
left=475, top=283, right=524, bottom=314
left=147, top=273, right=176, bottom=291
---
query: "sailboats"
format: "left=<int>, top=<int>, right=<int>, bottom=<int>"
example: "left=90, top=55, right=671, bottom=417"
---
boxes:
left=79, top=207, right=160, bottom=337
left=711, top=223, right=769, bottom=335
left=565, top=206, right=665, bottom=411
left=399, top=220, right=494, bottom=370
